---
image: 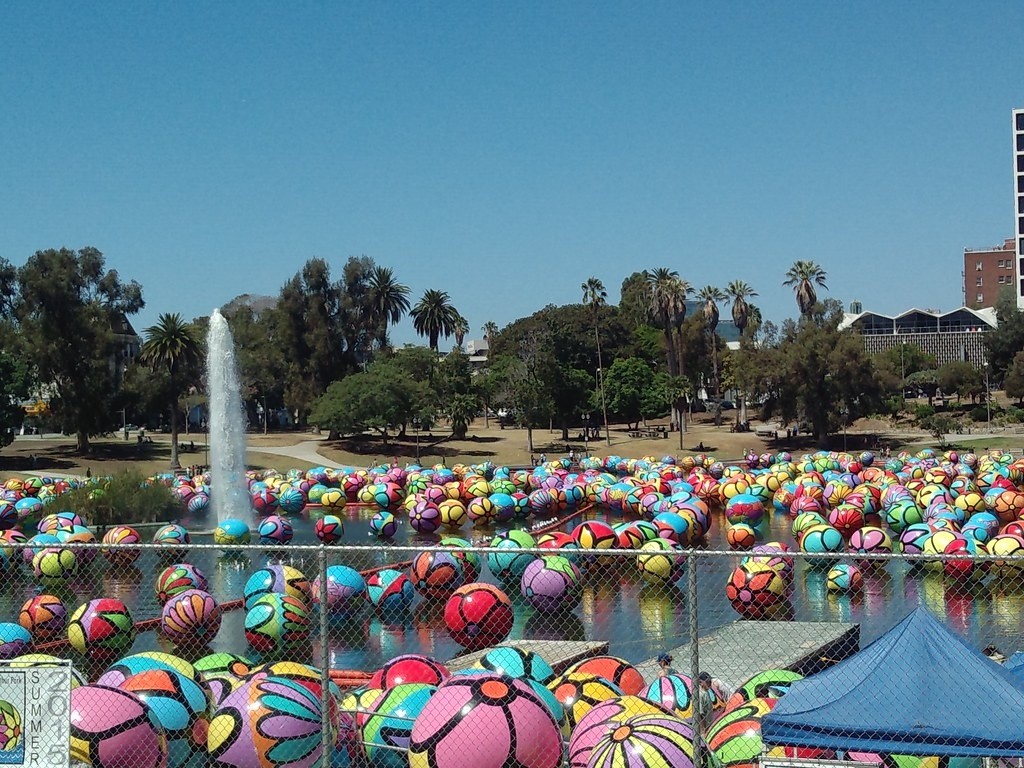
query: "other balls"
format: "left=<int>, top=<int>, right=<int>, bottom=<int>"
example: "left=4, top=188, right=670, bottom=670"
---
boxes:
left=0, top=448, right=1024, bottom=767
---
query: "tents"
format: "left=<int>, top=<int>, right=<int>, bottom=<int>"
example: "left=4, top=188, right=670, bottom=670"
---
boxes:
left=761, top=605, right=1024, bottom=768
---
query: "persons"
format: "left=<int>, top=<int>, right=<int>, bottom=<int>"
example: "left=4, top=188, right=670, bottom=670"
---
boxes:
left=368, top=458, right=377, bottom=471
left=138, top=436, right=151, bottom=444
left=406, top=456, right=420, bottom=467
left=775, top=425, right=798, bottom=442
left=969, top=445, right=974, bottom=453
left=187, top=464, right=202, bottom=478
left=730, top=421, right=750, bottom=432
left=877, top=437, right=880, bottom=444
left=863, top=436, right=869, bottom=443
left=30, top=454, right=37, bottom=463
left=566, top=444, right=582, bottom=461
left=743, top=448, right=755, bottom=460
left=442, top=456, right=445, bottom=464
left=579, top=429, right=599, bottom=439
left=393, top=456, right=399, bottom=466
left=879, top=446, right=891, bottom=459
left=696, top=442, right=703, bottom=451
left=715, top=414, right=721, bottom=428
left=656, top=654, right=679, bottom=677
left=191, top=440, right=194, bottom=449
left=530, top=453, right=546, bottom=466
left=87, top=467, right=91, bottom=478
left=698, top=671, right=734, bottom=733
